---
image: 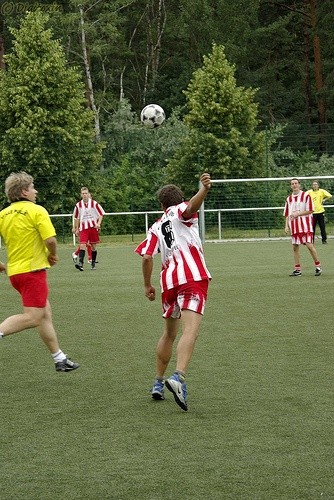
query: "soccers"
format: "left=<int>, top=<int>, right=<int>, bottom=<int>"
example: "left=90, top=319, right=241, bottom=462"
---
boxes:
left=141, top=104, right=166, bottom=128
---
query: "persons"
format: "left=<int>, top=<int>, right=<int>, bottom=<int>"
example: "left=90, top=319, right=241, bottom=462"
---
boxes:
left=0, top=172, right=80, bottom=371
left=306, top=181, right=332, bottom=244
left=72, top=187, right=105, bottom=271
left=134, top=173, right=212, bottom=411
left=283, top=178, right=322, bottom=276
